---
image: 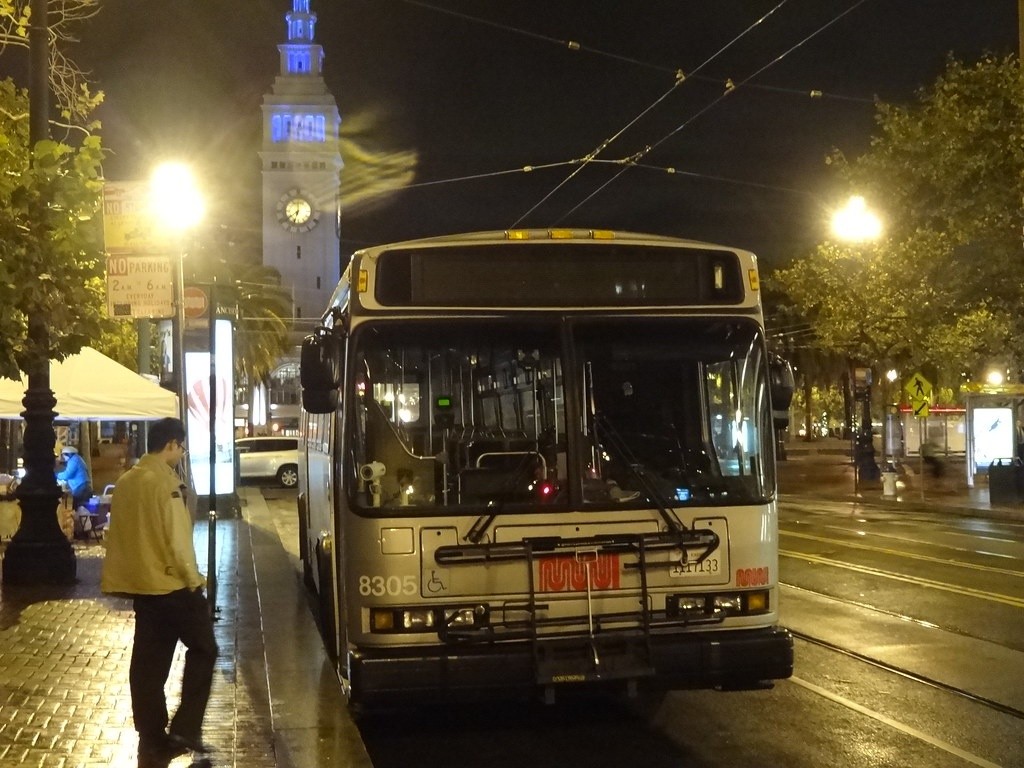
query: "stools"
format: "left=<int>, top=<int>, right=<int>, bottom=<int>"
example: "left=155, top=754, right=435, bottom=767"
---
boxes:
left=71, top=514, right=99, bottom=548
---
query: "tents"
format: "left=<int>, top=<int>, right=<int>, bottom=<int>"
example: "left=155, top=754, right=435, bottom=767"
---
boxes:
left=0, top=347, right=181, bottom=420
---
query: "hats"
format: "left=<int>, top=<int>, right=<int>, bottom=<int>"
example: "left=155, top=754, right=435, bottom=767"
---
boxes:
left=62, top=446, right=79, bottom=453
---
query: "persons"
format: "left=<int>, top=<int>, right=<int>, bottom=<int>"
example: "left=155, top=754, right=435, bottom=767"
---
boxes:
left=55, top=447, right=91, bottom=539
left=1016, top=420, right=1024, bottom=457
left=919, top=439, right=944, bottom=478
left=100, top=418, right=218, bottom=752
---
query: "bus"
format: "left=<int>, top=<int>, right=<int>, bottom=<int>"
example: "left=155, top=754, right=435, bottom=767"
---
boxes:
left=300, top=226, right=797, bottom=704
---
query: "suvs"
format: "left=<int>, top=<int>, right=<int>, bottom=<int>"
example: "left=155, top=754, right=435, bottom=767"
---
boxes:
left=233, top=437, right=300, bottom=489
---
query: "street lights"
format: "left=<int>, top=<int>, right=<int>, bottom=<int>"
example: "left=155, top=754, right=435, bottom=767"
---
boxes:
left=142, top=155, right=208, bottom=490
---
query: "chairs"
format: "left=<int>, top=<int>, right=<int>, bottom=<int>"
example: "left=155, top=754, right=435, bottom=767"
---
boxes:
left=427, top=426, right=533, bottom=472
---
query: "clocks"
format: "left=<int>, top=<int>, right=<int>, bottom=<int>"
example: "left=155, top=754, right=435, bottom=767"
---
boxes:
left=276, top=189, right=321, bottom=234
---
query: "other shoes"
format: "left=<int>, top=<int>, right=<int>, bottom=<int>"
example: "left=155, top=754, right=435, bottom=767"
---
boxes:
left=136, top=731, right=186, bottom=759
left=169, top=732, right=220, bottom=759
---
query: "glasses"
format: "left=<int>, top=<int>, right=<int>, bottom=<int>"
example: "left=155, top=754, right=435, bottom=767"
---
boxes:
left=175, top=442, right=189, bottom=455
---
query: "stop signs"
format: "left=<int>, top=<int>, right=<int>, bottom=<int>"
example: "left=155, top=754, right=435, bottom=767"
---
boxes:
left=180, top=287, right=208, bottom=319
